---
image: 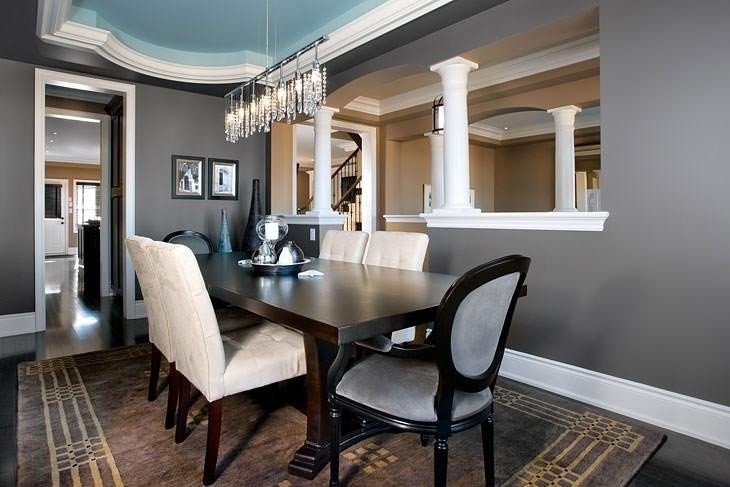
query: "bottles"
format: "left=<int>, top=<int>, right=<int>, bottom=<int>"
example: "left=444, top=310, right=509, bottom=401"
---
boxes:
left=278, top=238, right=304, bottom=264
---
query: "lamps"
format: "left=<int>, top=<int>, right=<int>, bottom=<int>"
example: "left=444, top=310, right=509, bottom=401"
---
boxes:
left=224, top=0, right=330, bottom=143
left=432, top=94, right=444, bottom=133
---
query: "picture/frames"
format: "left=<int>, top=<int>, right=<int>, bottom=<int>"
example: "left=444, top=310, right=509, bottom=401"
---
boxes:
left=172, top=155, right=239, bottom=200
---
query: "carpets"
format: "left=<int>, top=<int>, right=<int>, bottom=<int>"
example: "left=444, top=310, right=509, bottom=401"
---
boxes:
left=17, top=342, right=665, bottom=486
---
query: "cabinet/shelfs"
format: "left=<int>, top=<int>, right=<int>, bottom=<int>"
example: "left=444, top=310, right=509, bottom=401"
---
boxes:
left=78, top=224, right=99, bottom=300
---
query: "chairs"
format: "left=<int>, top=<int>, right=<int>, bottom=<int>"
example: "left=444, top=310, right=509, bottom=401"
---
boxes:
left=317, top=229, right=369, bottom=265
left=364, top=230, right=429, bottom=348
left=328, top=252, right=532, bottom=487
left=145, top=240, right=309, bottom=485
left=124, top=232, right=263, bottom=430
